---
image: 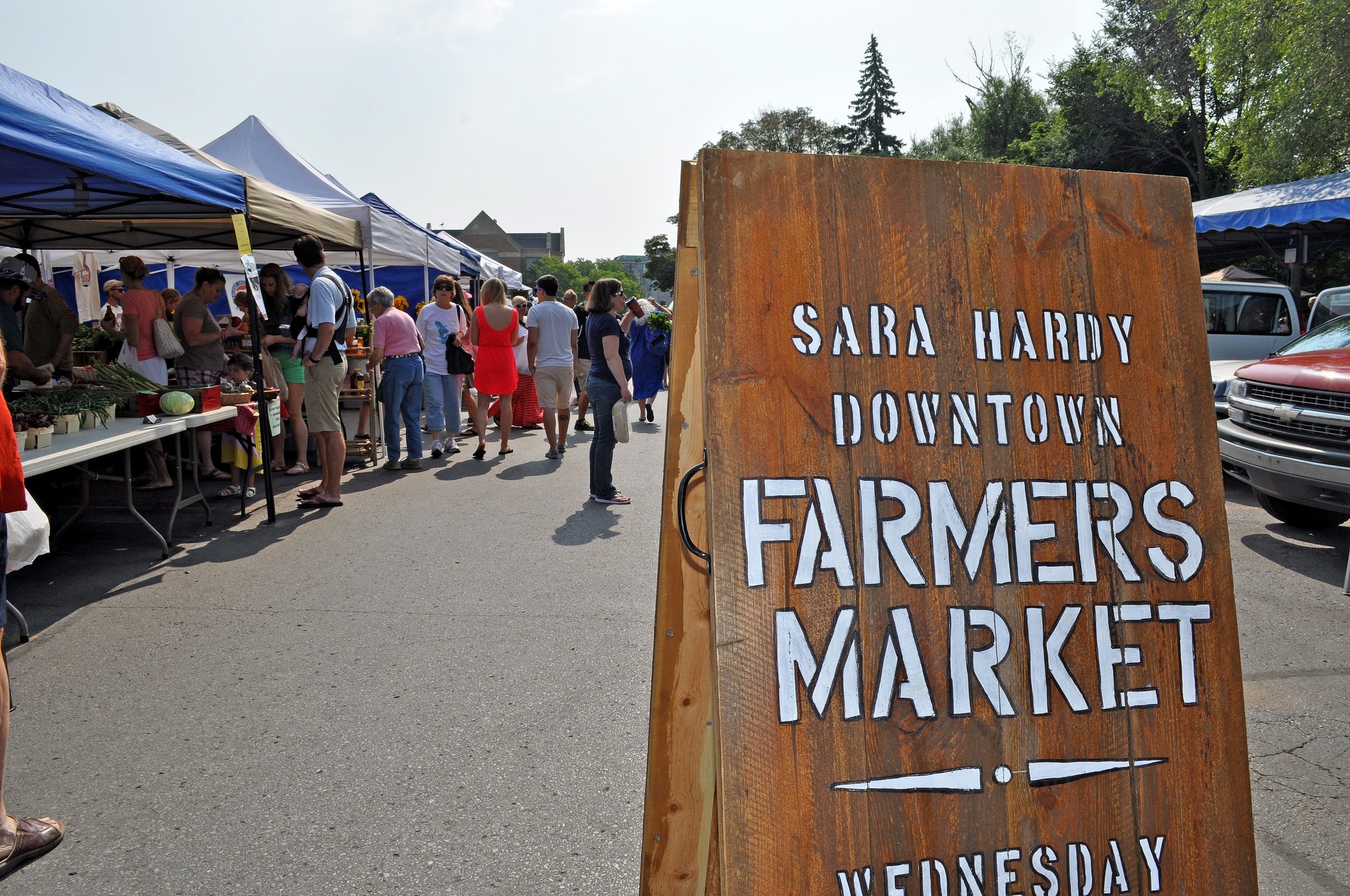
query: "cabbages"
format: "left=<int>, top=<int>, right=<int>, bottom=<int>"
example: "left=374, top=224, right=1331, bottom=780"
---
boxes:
left=159, top=391, right=195, bottom=415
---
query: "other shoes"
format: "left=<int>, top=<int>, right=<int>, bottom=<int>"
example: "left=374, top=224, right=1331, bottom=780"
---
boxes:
left=352, top=417, right=595, bottom=470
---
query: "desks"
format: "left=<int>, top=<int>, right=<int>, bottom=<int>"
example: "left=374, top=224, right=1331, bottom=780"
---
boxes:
left=19, top=396, right=282, bottom=559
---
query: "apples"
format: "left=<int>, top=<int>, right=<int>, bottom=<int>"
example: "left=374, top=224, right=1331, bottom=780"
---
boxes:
left=231, top=316, right=249, bottom=332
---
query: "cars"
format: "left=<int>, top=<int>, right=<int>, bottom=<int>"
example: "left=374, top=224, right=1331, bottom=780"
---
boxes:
left=1210, top=286, right=1350, bottom=531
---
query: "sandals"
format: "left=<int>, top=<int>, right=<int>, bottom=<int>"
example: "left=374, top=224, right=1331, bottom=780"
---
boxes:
left=216, top=485, right=256, bottom=497
left=271, top=460, right=284, bottom=471
left=285, top=462, right=310, bottom=476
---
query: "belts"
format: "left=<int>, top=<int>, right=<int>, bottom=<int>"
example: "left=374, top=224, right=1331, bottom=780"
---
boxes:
left=385, top=353, right=418, bottom=359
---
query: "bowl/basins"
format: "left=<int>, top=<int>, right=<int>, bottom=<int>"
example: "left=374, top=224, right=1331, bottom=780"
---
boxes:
left=72, top=367, right=100, bottom=379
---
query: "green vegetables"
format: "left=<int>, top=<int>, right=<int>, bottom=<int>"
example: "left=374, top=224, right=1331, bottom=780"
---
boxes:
left=71, top=324, right=126, bottom=351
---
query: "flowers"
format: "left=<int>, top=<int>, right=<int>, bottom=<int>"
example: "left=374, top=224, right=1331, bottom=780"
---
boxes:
left=416, top=300, right=433, bottom=316
left=394, top=296, right=409, bottom=311
left=351, top=289, right=366, bottom=314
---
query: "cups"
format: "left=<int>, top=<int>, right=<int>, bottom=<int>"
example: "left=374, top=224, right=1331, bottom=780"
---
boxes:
left=625, top=296, right=645, bottom=319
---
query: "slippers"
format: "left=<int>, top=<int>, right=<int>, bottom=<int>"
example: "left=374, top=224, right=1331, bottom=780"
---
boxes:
left=202, top=469, right=231, bottom=481
left=589, top=491, right=631, bottom=504
left=136, top=484, right=174, bottom=492
left=297, top=487, right=343, bottom=507
left=132, top=476, right=152, bottom=484
left=638, top=403, right=654, bottom=422
left=0, top=815, right=65, bottom=876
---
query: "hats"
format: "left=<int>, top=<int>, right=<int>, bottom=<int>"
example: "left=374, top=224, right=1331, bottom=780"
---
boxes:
left=0, top=256, right=48, bottom=300
left=103, top=279, right=124, bottom=292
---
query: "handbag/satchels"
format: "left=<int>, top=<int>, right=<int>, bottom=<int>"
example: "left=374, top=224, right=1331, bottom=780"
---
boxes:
left=645, top=323, right=668, bottom=356
left=152, top=290, right=185, bottom=358
left=117, top=338, right=143, bottom=376
left=261, top=346, right=288, bottom=402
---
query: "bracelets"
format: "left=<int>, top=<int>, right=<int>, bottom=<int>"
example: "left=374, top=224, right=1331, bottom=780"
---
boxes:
left=222, top=330, right=228, bottom=340
left=309, top=353, right=321, bottom=363
left=659, top=305, right=662, bottom=310
left=293, top=354, right=298, bottom=358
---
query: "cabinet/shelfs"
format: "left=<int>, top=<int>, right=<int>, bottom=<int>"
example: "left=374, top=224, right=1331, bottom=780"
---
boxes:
left=338, top=347, right=378, bottom=466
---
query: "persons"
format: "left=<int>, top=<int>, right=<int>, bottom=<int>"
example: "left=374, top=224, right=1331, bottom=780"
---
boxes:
left=0, top=232, right=675, bottom=878
left=1238, top=296, right=1345, bottom=331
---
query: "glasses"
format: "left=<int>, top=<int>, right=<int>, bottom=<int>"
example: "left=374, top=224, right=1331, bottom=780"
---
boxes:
left=111, top=288, right=124, bottom=293
left=516, top=304, right=527, bottom=308
left=584, top=289, right=592, bottom=293
left=434, top=284, right=453, bottom=291
left=612, top=290, right=623, bottom=297
left=119, top=257, right=126, bottom=269
left=533, top=286, right=546, bottom=293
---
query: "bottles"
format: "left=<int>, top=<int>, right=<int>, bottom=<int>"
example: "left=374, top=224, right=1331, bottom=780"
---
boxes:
left=357, top=337, right=364, bottom=354
left=348, top=368, right=365, bottom=396
left=104, top=305, right=116, bottom=328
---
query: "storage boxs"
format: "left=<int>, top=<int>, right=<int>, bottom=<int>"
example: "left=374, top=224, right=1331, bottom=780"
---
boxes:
left=7, top=385, right=221, bottom=453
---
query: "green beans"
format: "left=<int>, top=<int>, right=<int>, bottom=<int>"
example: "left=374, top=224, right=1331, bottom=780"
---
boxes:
left=5, top=385, right=126, bottom=430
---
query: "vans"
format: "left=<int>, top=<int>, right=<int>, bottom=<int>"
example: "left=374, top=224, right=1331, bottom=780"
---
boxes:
left=1198, top=278, right=1307, bottom=362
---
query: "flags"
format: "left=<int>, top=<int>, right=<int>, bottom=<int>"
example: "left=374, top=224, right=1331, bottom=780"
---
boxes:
left=1275, top=324, right=1289, bottom=333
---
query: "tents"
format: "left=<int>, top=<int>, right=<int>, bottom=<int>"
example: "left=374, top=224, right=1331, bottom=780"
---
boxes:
left=437, top=230, right=523, bottom=290
left=0, top=63, right=279, bottom=525
left=0, top=102, right=377, bottom=466
left=1191, top=171, right=1350, bottom=312
left=359, top=192, right=481, bottom=309
left=198, top=115, right=461, bottom=458
left=1200, top=265, right=1316, bottom=297
